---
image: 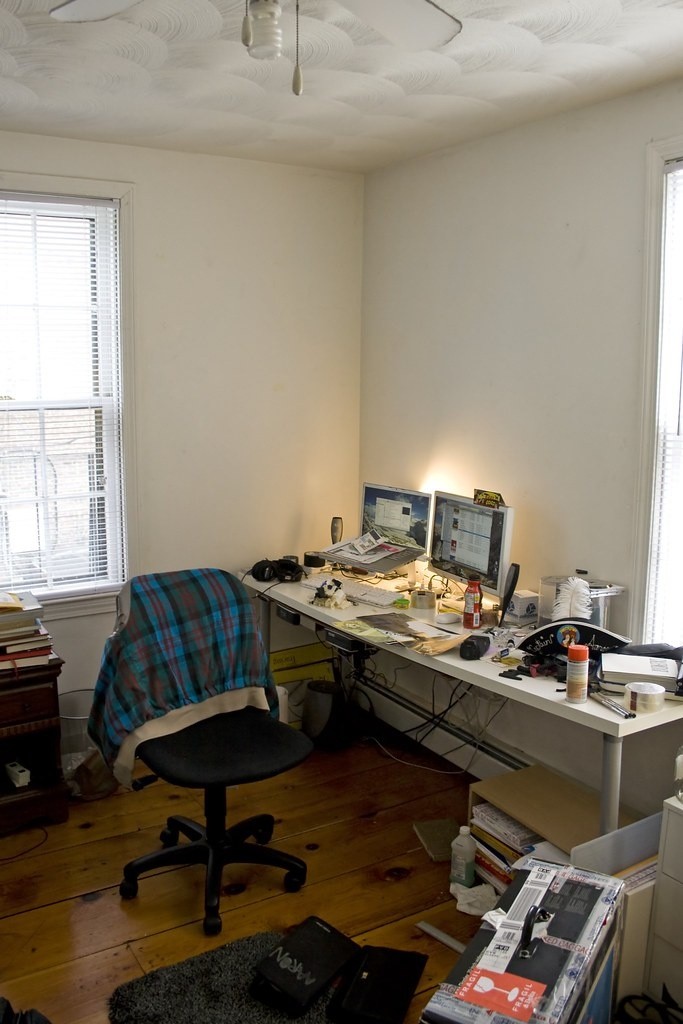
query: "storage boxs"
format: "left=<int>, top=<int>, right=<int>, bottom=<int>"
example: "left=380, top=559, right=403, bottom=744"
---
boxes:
left=467, top=766, right=650, bottom=862
left=507, top=590, right=539, bottom=621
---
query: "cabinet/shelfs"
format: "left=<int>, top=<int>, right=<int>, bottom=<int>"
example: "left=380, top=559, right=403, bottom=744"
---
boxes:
left=0, top=654, right=69, bottom=839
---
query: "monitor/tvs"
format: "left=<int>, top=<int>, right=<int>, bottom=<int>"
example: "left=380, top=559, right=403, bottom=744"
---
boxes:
left=429, top=491, right=514, bottom=614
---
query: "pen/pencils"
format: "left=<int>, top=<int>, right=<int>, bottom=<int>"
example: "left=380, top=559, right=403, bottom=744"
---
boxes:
left=588, top=691, right=637, bottom=719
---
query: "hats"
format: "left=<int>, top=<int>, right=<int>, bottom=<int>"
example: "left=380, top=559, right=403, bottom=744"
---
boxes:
left=514, top=576, right=632, bottom=662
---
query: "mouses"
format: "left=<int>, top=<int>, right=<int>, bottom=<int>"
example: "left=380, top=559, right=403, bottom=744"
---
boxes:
left=435, top=612, right=462, bottom=624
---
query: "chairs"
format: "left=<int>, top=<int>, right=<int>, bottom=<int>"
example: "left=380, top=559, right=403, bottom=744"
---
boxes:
left=106, top=568, right=307, bottom=938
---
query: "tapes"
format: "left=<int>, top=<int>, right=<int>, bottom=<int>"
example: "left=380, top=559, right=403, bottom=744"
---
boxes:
left=411, top=590, right=436, bottom=609
left=304, top=551, right=325, bottom=568
left=624, top=681, right=665, bottom=713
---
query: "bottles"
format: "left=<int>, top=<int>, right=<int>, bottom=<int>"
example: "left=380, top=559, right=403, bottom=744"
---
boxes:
left=566, top=645, right=589, bottom=705
left=448, top=826, right=476, bottom=888
left=463, top=573, right=482, bottom=629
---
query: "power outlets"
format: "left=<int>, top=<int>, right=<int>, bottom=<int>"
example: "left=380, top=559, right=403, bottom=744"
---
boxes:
left=479, top=687, right=503, bottom=700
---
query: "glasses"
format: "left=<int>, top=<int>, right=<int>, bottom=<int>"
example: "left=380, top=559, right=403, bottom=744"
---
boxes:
left=241, top=559, right=303, bottom=600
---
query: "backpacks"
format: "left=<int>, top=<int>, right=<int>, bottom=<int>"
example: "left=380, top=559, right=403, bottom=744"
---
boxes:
left=0, top=996, right=51, bottom=1024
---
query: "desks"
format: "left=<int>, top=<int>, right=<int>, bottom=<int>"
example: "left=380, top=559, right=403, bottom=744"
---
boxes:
left=237, top=564, right=683, bottom=834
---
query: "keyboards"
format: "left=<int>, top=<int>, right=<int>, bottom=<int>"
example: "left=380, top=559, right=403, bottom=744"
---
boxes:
left=300, top=573, right=404, bottom=609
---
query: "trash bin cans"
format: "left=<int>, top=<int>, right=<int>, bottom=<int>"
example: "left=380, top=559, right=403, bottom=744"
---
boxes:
left=59, top=688, right=122, bottom=798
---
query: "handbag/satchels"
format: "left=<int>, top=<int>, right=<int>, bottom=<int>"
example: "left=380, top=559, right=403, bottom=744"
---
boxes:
left=618, top=643, right=683, bottom=660
left=327, top=944, right=430, bottom=1024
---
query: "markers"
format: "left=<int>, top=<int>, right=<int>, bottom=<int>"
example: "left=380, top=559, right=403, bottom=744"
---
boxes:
left=345, top=566, right=368, bottom=574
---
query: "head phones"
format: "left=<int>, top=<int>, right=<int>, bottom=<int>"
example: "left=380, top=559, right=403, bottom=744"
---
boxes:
left=250, top=558, right=303, bottom=582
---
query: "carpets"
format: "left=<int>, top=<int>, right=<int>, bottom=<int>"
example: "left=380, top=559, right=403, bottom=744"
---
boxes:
left=109, top=932, right=335, bottom=1024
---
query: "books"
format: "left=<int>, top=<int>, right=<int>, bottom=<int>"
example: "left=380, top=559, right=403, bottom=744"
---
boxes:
left=0, top=591, right=53, bottom=669
left=470, top=803, right=543, bottom=898
left=602, top=652, right=677, bottom=692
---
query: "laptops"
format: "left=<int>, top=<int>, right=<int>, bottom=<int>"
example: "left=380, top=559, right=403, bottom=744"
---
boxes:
left=318, top=481, right=432, bottom=575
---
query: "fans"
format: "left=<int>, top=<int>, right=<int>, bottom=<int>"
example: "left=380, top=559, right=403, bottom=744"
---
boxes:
left=49, top=0, right=461, bottom=51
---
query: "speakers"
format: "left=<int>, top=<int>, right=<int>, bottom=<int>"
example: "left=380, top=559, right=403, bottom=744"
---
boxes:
left=496, top=563, right=521, bottom=627
left=300, top=680, right=355, bottom=754
left=331, top=516, right=343, bottom=544
